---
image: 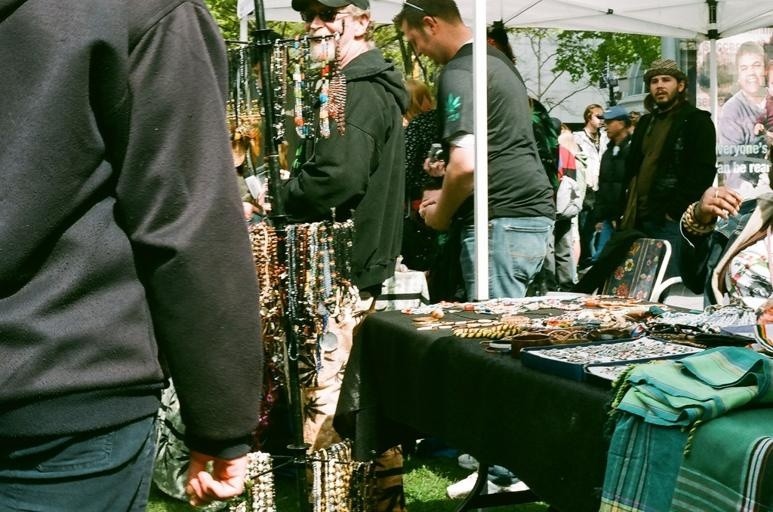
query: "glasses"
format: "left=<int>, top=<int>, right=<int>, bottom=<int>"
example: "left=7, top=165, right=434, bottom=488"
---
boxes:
left=300, top=8, right=352, bottom=22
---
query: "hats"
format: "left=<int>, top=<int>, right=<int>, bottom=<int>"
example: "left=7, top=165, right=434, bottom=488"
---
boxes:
left=291, top=0, right=370, bottom=10
left=643, top=59, right=688, bottom=85
left=597, top=106, right=631, bottom=120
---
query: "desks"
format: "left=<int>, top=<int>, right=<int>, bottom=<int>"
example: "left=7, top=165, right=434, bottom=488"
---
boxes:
left=348, top=288, right=772, bottom=512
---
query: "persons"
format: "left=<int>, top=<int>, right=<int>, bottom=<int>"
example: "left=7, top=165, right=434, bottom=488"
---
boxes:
left=251, top=0, right=773, bottom=458
left=1, top=0, right=264, bottom=512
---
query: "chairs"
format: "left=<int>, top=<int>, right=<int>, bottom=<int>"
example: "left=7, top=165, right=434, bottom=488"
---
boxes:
left=591, top=235, right=684, bottom=306
